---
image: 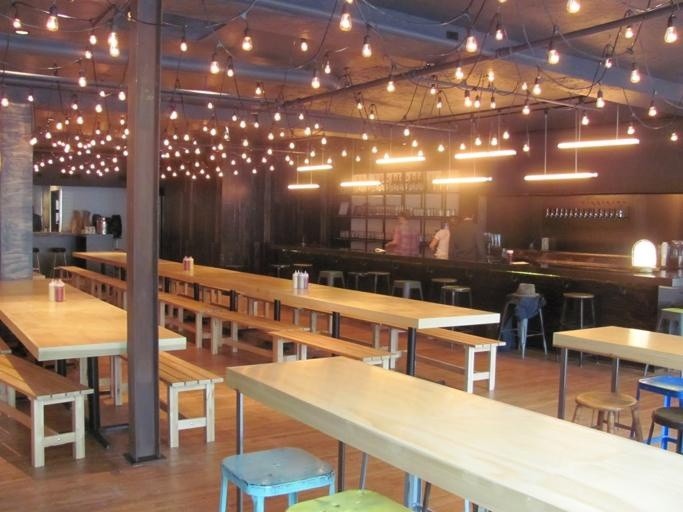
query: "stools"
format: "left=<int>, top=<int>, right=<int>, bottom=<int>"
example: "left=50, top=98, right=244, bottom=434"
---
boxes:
left=219, top=445, right=336, bottom=512
left=358, top=452, right=423, bottom=509
left=283, top=489, right=418, bottom=511
left=0, top=246, right=681, bottom=469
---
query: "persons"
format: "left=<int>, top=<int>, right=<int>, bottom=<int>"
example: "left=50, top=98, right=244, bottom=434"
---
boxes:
left=384, top=210, right=421, bottom=258
left=447, top=205, right=487, bottom=263
left=426, top=217, right=451, bottom=261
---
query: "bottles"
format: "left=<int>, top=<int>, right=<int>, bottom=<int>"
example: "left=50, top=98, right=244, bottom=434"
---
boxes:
left=183, top=255, right=194, bottom=271
left=292, top=270, right=309, bottom=289
left=354, top=172, right=449, bottom=193
left=71, top=209, right=105, bottom=236
left=48, top=278, right=66, bottom=302
left=353, top=204, right=410, bottom=217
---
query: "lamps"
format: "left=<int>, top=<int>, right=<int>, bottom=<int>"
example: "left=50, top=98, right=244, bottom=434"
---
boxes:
left=157, top=2, right=683, bottom=191
left=1, top=1, right=132, bottom=178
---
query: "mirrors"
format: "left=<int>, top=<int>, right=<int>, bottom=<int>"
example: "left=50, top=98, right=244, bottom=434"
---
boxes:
left=33, top=183, right=62, bottom=232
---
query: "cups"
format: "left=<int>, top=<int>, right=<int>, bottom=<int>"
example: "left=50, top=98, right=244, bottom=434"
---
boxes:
left=541, top=237, right=550, bottom=250
left=412, top=207, right=456, bottom=216
left=338, top=230, right=422, bottom=242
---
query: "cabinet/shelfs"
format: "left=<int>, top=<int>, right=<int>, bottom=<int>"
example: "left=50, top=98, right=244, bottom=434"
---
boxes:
left=335, top=189, right=460, bottom=257
